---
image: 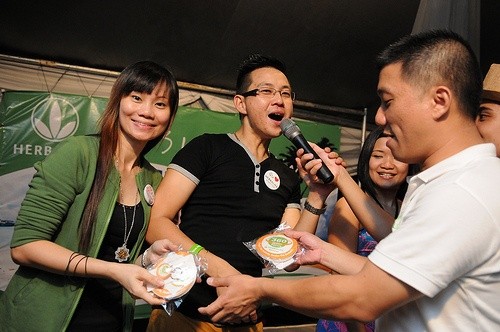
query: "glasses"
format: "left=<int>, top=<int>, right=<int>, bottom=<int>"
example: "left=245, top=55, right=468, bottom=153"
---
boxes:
left=241, top=88, right=296, bottom=101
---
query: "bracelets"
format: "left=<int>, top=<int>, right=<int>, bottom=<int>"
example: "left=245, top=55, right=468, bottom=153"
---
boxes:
left=65, top=252, right=89, bottom=275
left=142, top=249, right=148, bottom=267
left=304, top=199, right=327, bottom=215
left=189, top=244, right=204, bottom=256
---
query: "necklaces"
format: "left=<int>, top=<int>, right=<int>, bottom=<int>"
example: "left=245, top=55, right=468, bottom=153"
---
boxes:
left=115, top=191, right=138, bottom=262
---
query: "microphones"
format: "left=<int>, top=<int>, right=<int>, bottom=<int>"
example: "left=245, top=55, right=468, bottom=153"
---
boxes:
left=281, top=118, right=334, bottom=183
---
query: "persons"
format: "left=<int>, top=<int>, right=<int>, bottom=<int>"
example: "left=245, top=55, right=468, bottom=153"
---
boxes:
left=475, top=63, right=500, bottom=157
left=0, top=60, right=202, bottom=332
left=145, top=50, right=346, bottom=332
left=197, top=28, right=500, bottom=332
left=316, top=127, right=415, bottom=332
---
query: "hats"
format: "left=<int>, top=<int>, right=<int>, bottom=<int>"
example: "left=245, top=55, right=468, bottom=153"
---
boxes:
left=482, top=63, right=500, bottom=105
